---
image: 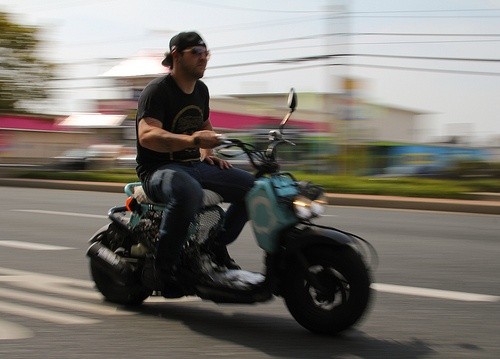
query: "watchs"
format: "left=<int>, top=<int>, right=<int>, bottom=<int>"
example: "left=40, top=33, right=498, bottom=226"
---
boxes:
left=190, top=135, right=200, bottom=149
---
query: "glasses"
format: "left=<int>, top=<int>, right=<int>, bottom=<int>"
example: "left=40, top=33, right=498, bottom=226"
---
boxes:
left=175, top=47, right=212, bottom=57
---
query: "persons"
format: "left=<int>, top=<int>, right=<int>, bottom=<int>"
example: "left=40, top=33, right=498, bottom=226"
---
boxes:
left=135, top=30, right=255, bottom=287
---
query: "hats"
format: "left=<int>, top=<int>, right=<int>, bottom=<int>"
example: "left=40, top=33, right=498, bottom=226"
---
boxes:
left=162, top=32, right=207, bottom=67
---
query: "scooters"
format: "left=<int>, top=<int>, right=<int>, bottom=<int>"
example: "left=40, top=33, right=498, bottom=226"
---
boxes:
left=86, top=88, right=379, bottom=337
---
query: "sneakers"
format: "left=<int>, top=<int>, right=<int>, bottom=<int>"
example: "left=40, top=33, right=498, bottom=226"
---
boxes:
left=152, top=251, right=183, bottom=299
left=201, top=238, right=242, bottom=271
